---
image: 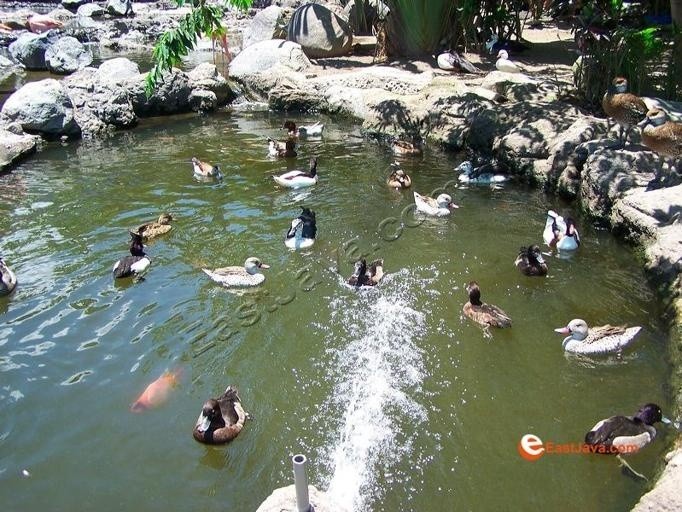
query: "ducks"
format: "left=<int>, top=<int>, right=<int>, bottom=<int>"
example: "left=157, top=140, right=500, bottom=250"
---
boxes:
left=0, top=40, right=681, bottom=482
